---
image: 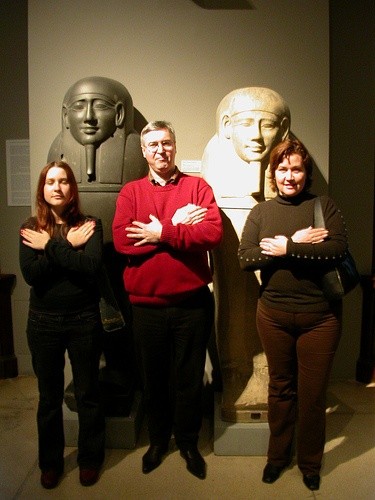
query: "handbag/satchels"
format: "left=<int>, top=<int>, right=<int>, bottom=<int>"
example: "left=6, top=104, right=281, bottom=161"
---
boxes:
left=314, top=195, right=361, bottom=294
left=86, top=215, right=126, bottom=332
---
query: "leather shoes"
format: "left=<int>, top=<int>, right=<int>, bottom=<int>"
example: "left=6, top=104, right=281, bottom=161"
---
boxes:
left=262, top=461, right=287, bottom=484
left=302, top=472, right=321, bottom=490
left=141, top=441, right=169, bottom=474
left=180, top=447, right=206, bottom=479
left=41, top=467, right=61, bottom=489
left=79, top=460, right=99, bottom=487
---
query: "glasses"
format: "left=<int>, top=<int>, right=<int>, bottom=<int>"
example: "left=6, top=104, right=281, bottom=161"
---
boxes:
left=142, top=140, right=177, bottom=152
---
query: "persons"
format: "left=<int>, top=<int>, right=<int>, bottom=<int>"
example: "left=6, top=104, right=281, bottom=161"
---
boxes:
left=111, top=119, right=222, bottom=479
left=238, top=136, right=349, bottom=490
left=200, top=85, right=308, bottom=423
left=44, top=78, right=146, bottom=416
left=16, top=160, right=112, bottom=488
